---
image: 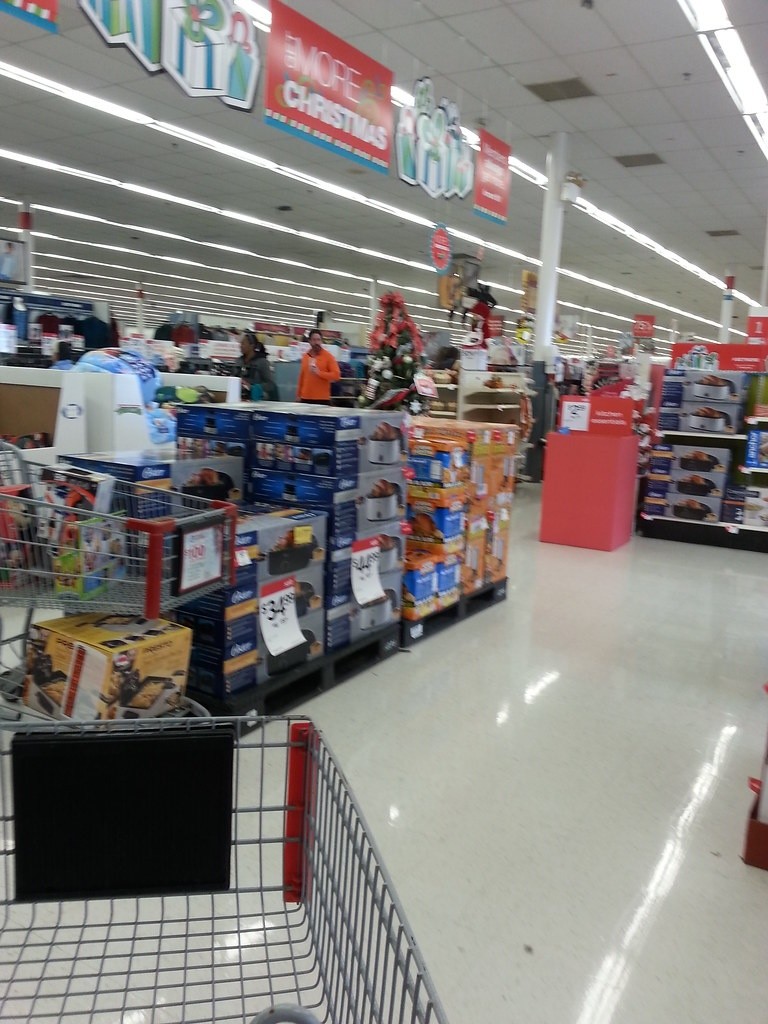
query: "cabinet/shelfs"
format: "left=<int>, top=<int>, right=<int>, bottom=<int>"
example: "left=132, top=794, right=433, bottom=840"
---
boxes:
left=0, top=285, right=643, bottom=482
left=636, top=369, right=767, bottom=553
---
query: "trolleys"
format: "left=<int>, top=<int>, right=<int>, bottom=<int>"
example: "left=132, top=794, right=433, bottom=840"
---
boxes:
left=1, top=438, right=239, bottom=717
left=0, top=712, right=448, bottom=1024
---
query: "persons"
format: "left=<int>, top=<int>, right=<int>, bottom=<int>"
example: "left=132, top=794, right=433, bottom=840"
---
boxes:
left=0, top=242, right=16, bottom=280
left=230, top=333, right=279, bottom=401
left=297, top=329, right=340, bottom=406
left=433, top=346, right=459, bottom=370
left=49, top=341, right=75, bottom=370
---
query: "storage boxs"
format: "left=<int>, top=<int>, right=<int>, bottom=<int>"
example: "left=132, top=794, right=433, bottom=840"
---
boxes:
left=175, top=400, right=520, bottom=649
left=168, top=565, right=325, bottom=646
left=185, top=611, right=324, bottom=698
left=55, top=450, right=244, bottom=508
left=221, top=501, right=328, bottom=578
left=0, top=460, right=129, bottom=602
left=644, top=368, right=768, bottom=532
left=20, top=613, right=191, bottom=719
